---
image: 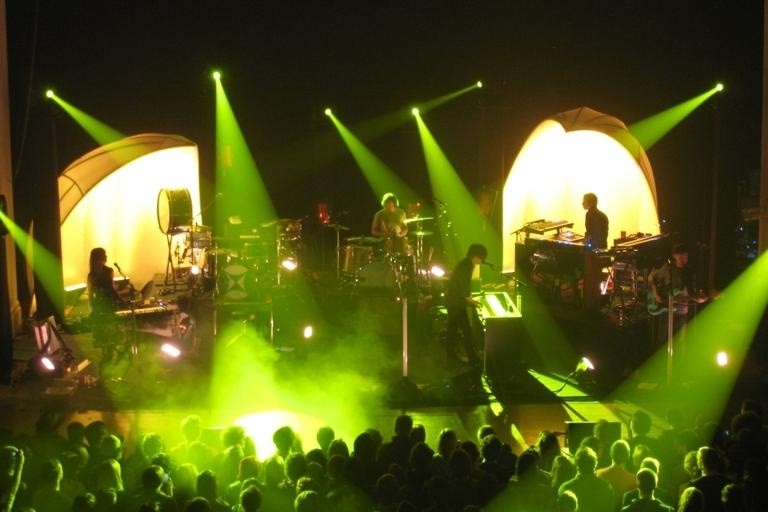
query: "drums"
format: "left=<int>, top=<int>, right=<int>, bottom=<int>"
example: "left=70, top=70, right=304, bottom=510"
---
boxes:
left=215, top=262, right=257, bottom=302
left=182, top=230, right=215, bottom=250
left=158, top=189, right=193, bottom=234
left=342, top=243, right=373, bottom=277
left=353, top=261, right=396, bottom=289
left=386, top=235, right=413, bottom=256
left=203, top=249, right=230, bottom=280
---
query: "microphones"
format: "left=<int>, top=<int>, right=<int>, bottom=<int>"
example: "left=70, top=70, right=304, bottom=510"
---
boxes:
left=432, top=198, right=447, bottom=207
left=482, top=259, right=496, bottom=267
left=667, top=256, right=671, bottom=264
left=114, top=263, right=121, bottom=272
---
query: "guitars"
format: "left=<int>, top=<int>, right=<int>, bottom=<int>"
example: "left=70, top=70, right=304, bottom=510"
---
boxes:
left=647, top=288, right=719, bottom=315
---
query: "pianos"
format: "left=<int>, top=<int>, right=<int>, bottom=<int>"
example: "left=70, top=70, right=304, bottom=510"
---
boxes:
left=112, top=306, right=179, bottom=320
left=607, top=234, right=671, bottom=257
left=470, top=296, right=505, bottom=329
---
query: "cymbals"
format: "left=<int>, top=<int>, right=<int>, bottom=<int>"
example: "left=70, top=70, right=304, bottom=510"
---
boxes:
left=408, top=230, right=433, bottom=236
left=345, top=236, right=383, bottom=243
left=326, top=224, right=352, bottom=232
left=402, top=217, right=433, bottom=224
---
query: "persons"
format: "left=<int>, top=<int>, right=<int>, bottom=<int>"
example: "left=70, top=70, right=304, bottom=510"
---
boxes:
left=582, top=193, right=608, bottom=249
left=372, top=192, right=409, bottom=256
left=88, top=248, right=129, bottom=360
left=1, top=399, right=768, bottom=511
left=444, top=245, right=487, bottom=364
left=647, top=243, right=692, bottom=352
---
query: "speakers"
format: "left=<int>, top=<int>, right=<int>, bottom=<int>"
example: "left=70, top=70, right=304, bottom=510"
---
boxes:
left=565, top=422, right=621, bottom=454
